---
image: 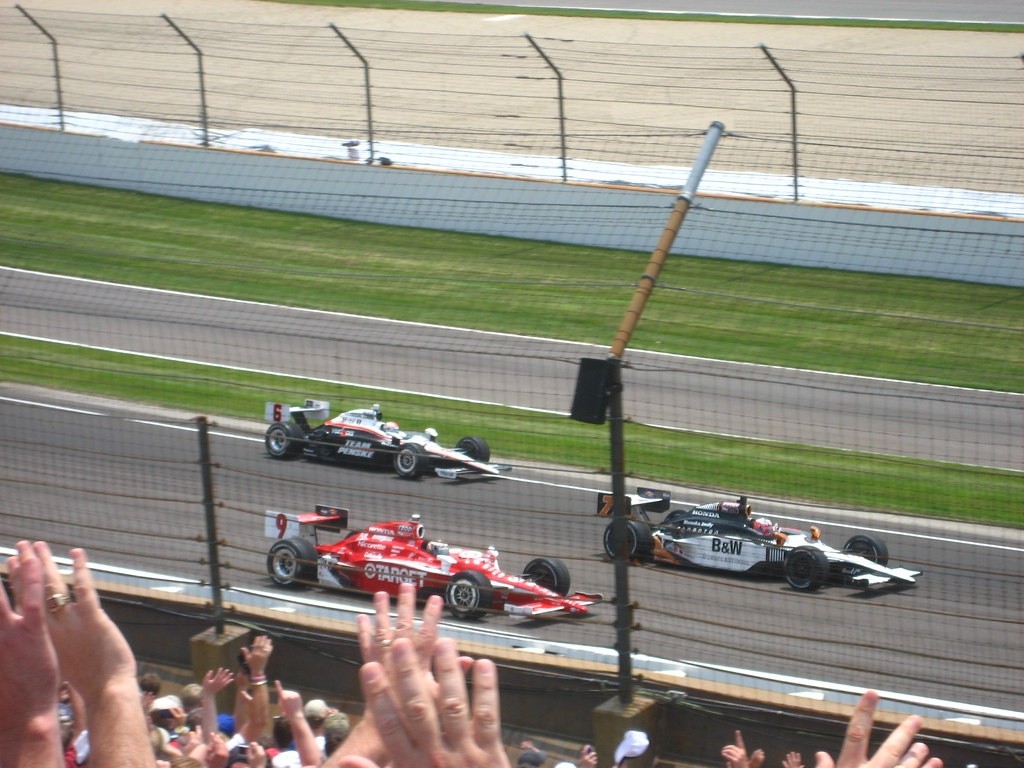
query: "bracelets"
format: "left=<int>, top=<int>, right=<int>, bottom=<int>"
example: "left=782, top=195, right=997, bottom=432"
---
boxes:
left=250, top=680, right=267, bottom=685
left=249, top=675, right=266, bottom=681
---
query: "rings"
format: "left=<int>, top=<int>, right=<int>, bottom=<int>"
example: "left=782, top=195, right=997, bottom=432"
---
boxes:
left=46, top=593, right=71, bottom=612
left=376, top=639, right=392, bottom=647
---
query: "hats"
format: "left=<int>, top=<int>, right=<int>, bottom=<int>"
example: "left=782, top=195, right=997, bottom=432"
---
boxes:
left=304, top=700, right=328, bottom=717
left=149, top=695, right=181, bottom=712
left=266, top=747, right=278, bottom=759
left=272, top=751, right=301, bottom=768
left=181, top=683, right=203, bottom=705
left=226, top=734, right=250, bottom=751
left=324, top=712, right=350, bottom=736
left=519, top=750, right=547, bottom=765
left=218, top=714, right=235, bottom=734
left=316, top=737, right=327, bottom=752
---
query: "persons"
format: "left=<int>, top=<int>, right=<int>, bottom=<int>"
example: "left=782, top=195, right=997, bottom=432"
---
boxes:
left=0, top=539, right=514, bottom=768
left=754, top=518, right=774, bottom=535
left=517, top=730, right=665, bottom=768
left=720, top=690, right=944, bottom=768
left=426, top=540, right=450, bottom=555
left=382, top=421, right=399, bottom=433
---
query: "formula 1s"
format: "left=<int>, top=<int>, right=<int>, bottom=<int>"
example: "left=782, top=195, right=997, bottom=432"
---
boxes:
left=264, top=399, right=513, bottom=481
left=265, top=503, right=605, bottom=627
left=596, top=487, right=925, bottom=591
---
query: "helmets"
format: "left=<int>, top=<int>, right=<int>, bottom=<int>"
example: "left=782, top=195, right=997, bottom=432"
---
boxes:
left=425, top=539, right=449, bottom=554
left=754, top=518, right=772, bottom=535
left=383, top=422, right=399, bottom=431
